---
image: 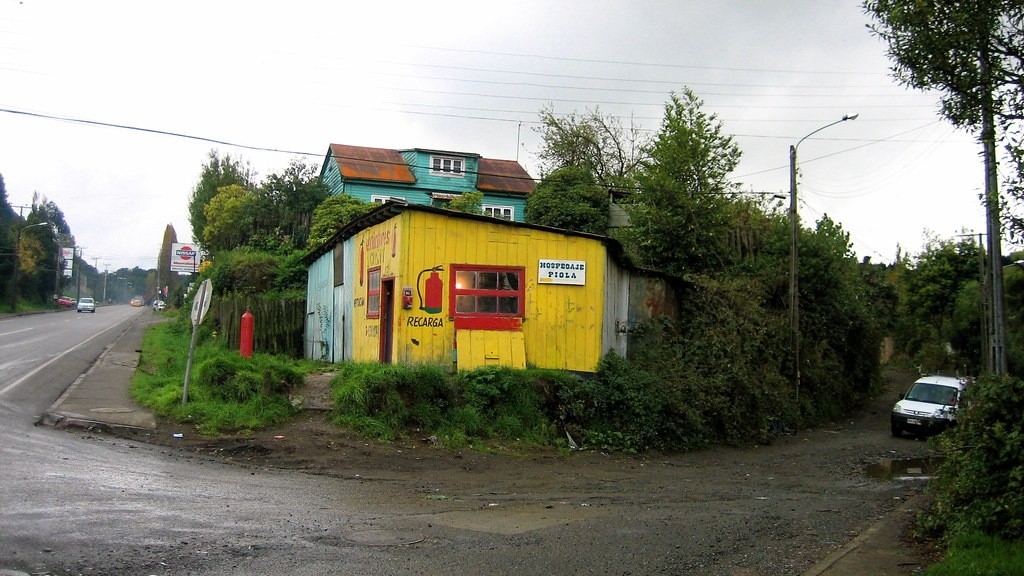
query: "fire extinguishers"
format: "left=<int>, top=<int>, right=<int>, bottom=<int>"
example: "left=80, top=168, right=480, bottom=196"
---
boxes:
left=239, top=306, right=255, bottom=359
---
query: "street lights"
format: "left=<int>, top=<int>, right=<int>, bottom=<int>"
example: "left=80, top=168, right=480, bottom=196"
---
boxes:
left=790, top=112, right=860, bottom=406
left=958, top=233, right=1006, bottom=372
left=984, top=259, right=1024, bottom=372
left=11, top=222, right=48, bottom=313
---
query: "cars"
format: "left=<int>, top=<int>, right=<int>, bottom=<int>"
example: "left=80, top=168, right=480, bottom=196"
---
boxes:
left=77, top=298, right=96, bottom=312
left=57, top=296, right=72, bottom=307
left=71, top=298, right=77, bottom=305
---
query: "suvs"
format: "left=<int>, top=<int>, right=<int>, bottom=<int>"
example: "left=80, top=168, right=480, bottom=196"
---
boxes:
left=153, top=300, right=166, bottom=312
left=891, top=376, right=968, bottom=440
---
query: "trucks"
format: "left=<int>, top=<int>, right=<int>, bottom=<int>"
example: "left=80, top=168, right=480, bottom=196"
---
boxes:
left=132, top=295, right=142, bottom=307
